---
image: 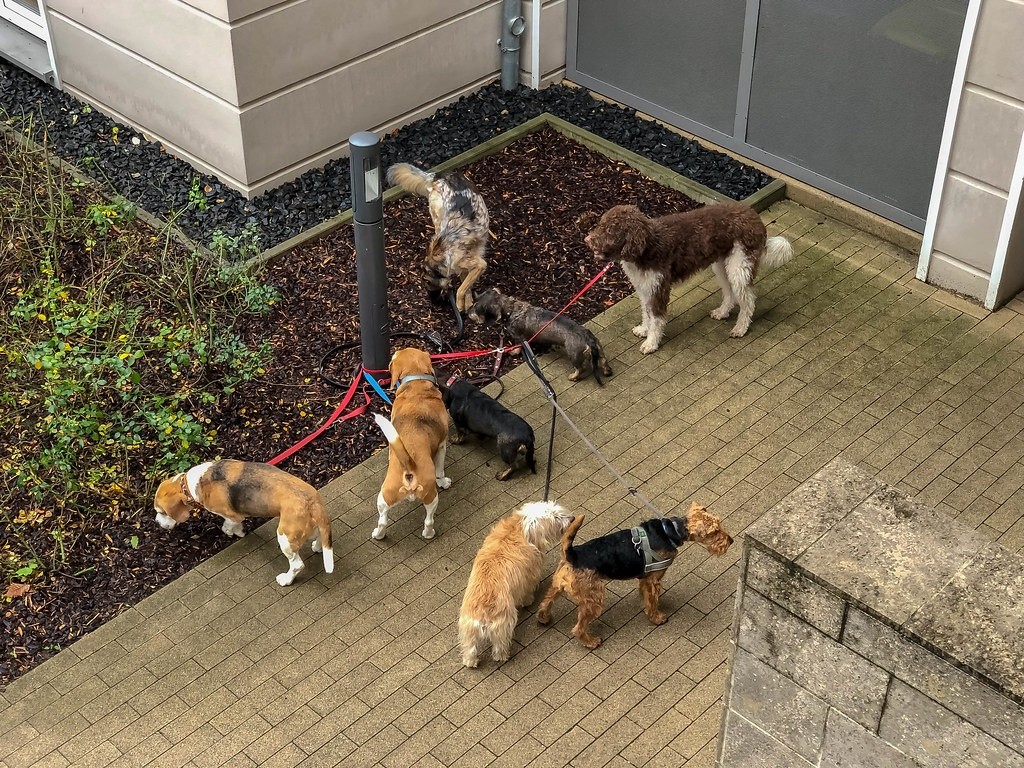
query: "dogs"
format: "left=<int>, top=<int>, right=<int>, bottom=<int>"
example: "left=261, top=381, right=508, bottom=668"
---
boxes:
left=153, top=459, right=333, bottom=586
left=372, top=348, right=452, bottom=540
left=584, top=204, right=794, bottom=354
left=536, top=501, right=734, bottom=649
left=468, top=287, right=614, bottom=385
left=436, top=368, right=537, bottom=481
left=387, top=163, right=489, bottom=311
left=458, top=500, right=576, bottom=668
